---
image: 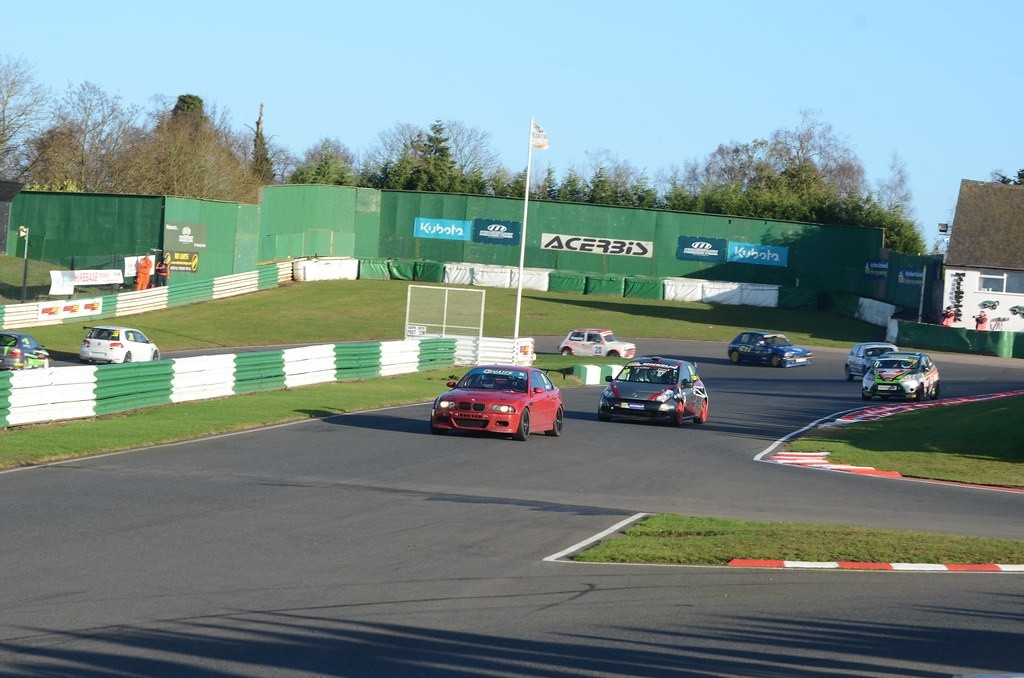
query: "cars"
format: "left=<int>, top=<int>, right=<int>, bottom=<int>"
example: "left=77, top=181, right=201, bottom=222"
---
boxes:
left=843, top=341, right=900, bottom=381
left=728, top=330, right=812, bottom=369
left=558, top=328, right=637, bottom=359
left=78, top=326, right=161, bottom=364
left=0, top=331, right=50, bottom=371
left=598, top=356, right=709, bottom=426
left=861, top=349, right=940, bottom=402
left=428, top=363, right=566, bottom=442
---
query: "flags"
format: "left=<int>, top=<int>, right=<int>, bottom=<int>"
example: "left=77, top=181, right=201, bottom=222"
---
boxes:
left=532, top=122, right=549, bottom=148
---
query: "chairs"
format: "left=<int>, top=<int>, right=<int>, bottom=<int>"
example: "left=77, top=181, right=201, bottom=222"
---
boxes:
left=632, top=369, right=654, bottom=382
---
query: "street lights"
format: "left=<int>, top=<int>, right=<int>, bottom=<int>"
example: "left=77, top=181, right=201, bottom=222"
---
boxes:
left=19, top=225, right=29, bottom=303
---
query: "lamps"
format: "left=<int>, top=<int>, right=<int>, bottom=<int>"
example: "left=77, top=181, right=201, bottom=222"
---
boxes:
left=938, top=223, right=953, bottom=232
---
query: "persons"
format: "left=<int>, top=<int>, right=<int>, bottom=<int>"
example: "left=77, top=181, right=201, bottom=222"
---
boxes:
left=756, top=336, right=766, bottom=346
left=940, top=306, right=955, bottom=326
left=975, top=311, right=988, bottom=330
left=480, top=375, right=496, bottom=388
left=156, top=259, right=170, bottom=287
left=137, top=252, right=152, bottom=290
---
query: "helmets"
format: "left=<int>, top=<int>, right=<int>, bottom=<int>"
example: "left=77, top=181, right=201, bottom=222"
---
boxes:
left=900, top=361, right=913, bottom=369
left=509, top=376, right=526, bottom=392
left=480, top=374, right=496, bottom=388
